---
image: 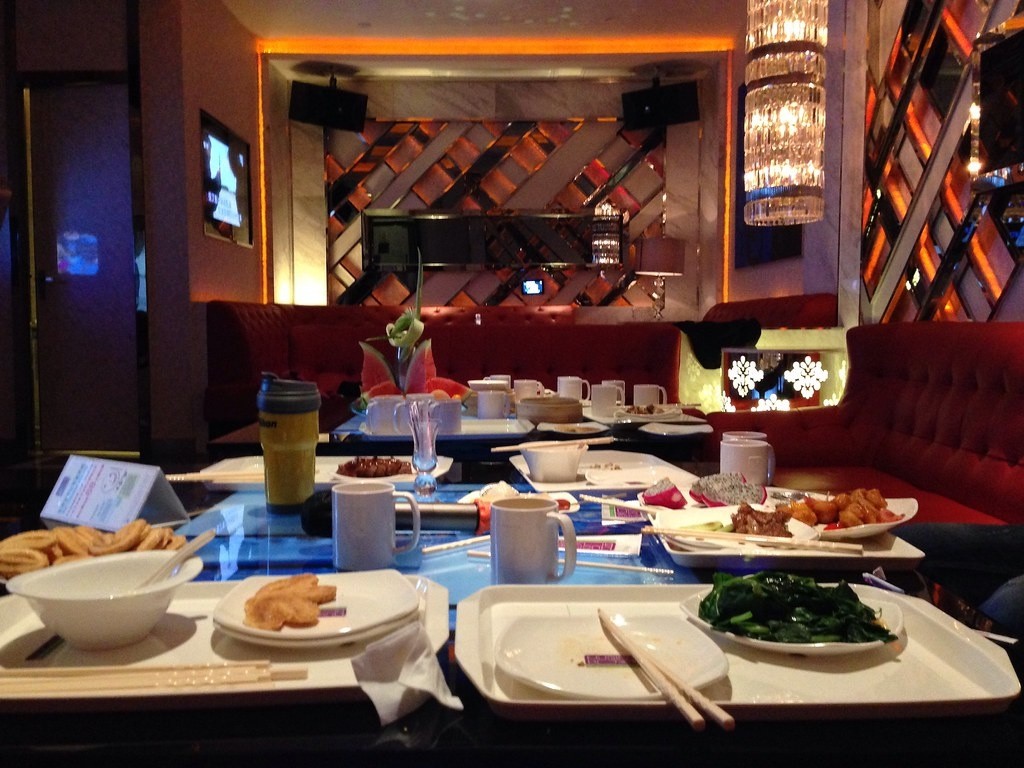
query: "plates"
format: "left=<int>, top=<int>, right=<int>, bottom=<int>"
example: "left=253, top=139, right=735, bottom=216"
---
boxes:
left=494, top=615, right=730, bottom=701
left=212, top=568, right=421, bottom=651
left=327, top=464, right=416, bottom=482
left=508, top=451, right=699, bottom=492
left=537, top=398, right=714, bottom=436
left=678, top=587, right=905, bottom=657
left=637, top=489, right=919, bottom=552
left=585, top=465, right=687, bottom=486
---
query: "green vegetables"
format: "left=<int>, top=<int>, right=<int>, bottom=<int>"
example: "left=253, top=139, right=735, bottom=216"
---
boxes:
left=696, top=568, right=900, bottom=645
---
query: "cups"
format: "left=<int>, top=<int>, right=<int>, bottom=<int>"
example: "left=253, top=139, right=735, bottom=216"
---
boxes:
left=365, top=392, right=462, bottom=434
left=469, top=374, right=626, bottom=420
left=331, top=483, right=422, bottom=571
left=634, top=384, right=668, bottom=406
left=490, top=497, right=577, bottom=586
left=256, top=373, right=321, bottom=515
left=720, top=431, right=774, bottom=486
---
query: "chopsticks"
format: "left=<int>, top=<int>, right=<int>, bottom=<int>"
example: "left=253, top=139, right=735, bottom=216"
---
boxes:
left=579, top=493, right=657, bottom=516
left=641, top=524, right=864, bottom=555
left=597, top=607, right=736, bottom=731
left=424, top=531, right=674, bottom=576
left=491, top=436, right=614, bottom=452
left=0, top=659, right=309, bottom=699
left=163, top=472, right=265, bottom=481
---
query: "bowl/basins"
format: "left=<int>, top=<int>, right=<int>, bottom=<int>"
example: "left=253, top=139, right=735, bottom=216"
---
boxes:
left=5, top=549, right=204, bottom=652
left=519, top=441, right=589, bottom=483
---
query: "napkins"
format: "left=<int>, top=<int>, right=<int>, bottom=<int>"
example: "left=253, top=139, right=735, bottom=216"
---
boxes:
left=352, top=621, right=463, bottom=727
left=638, top=421, right=714, bottom=437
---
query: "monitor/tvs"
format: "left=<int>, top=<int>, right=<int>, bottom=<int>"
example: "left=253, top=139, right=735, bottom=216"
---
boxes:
left=200, top=113, right=251, bottom=244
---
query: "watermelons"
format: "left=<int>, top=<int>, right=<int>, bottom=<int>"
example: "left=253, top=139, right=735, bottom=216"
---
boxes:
left=359, top=337, right=473, bottom=410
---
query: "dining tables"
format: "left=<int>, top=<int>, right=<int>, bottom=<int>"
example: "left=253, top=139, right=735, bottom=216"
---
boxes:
left=0, top=393, right=1024, bottom=768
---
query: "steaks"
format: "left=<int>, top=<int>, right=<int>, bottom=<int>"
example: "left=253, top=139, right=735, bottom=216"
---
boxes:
left=336, top=457, right=411, bottom=477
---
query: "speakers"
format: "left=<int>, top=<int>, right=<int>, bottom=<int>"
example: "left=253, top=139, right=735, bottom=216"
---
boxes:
left=621, top=81, right=700, bottom=132
left=288, top=80, right=368, bottom=134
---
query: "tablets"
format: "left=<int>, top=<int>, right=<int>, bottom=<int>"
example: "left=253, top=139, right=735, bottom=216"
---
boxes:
left=523, top=280, right=544, bottom=295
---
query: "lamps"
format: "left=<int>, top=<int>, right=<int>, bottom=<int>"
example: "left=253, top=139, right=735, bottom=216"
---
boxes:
left=743, top=0, right=828, bottom=227
left=634, top=237, right=686, bottom=321
left=594, top=199, right=621, bottom=266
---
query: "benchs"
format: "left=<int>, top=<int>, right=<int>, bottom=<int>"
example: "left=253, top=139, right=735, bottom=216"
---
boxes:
left=703, top=318, right=1023, bottom=526
left=203, top=299, right=687, bottom=422
left=702, top=293, right=840, bottom=327
